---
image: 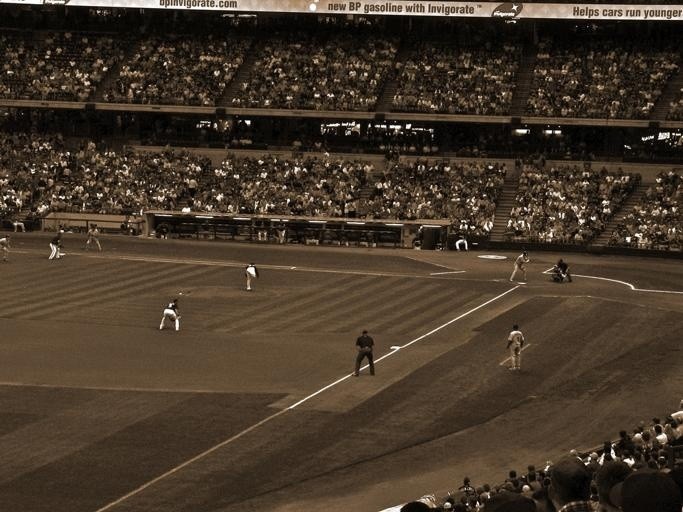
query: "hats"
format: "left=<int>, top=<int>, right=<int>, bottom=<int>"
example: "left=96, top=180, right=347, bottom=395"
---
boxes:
left=609, top=469, right=683, bottom=512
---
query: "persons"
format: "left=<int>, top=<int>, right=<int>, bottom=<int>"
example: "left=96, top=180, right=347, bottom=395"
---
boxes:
left=1, top=3, right=683, bottom=257
left=551, top=259, right=571, bottom=282
left=351, top=330, right=375, bottom=377
left=0, top=234, right=12, bottom=264
left=504, top=323, right=525, bottom=371
left=508, top=251, right=529, bottom=282
left=46, top=231, right=63, bottom=261
left=158, top=298, right=181, bottom=332
left=391, top=410, right=683, bottom=512
left=243, top=261, right=258, bottom=291
